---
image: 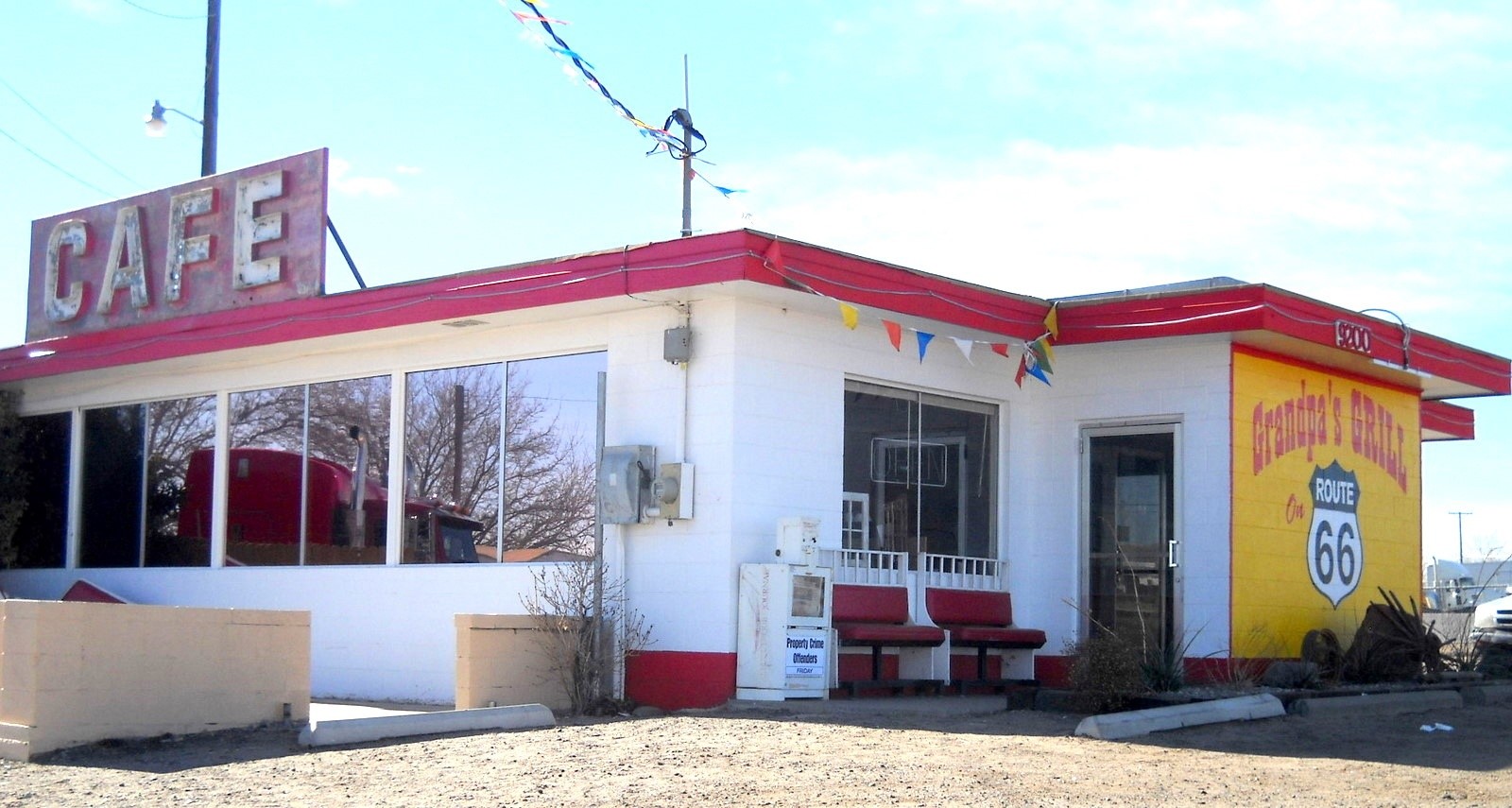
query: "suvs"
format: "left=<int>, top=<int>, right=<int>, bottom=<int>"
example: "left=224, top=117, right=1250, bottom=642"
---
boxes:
left=1469, top=585, right=1512, bottom=662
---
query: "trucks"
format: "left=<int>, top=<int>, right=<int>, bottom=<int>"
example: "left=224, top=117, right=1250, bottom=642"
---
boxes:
left=1423, top=556, right=1480, bottom=612
left=178, top=424, right=485, bottom=564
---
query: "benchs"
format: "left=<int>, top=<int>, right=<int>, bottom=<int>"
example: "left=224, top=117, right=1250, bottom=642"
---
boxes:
left=916, top=552, right=1047, bottom=684
left=821, top=548, right=946, bottom=696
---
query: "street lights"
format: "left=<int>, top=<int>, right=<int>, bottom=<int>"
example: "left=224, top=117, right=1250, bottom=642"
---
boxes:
left=145, top=98, right=218, bottom=178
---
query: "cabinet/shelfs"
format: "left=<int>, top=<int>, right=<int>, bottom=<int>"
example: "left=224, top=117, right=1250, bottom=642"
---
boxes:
left=735, top=563, right=839, bottom=702
left=842, top=492, right=869, bottom=566
